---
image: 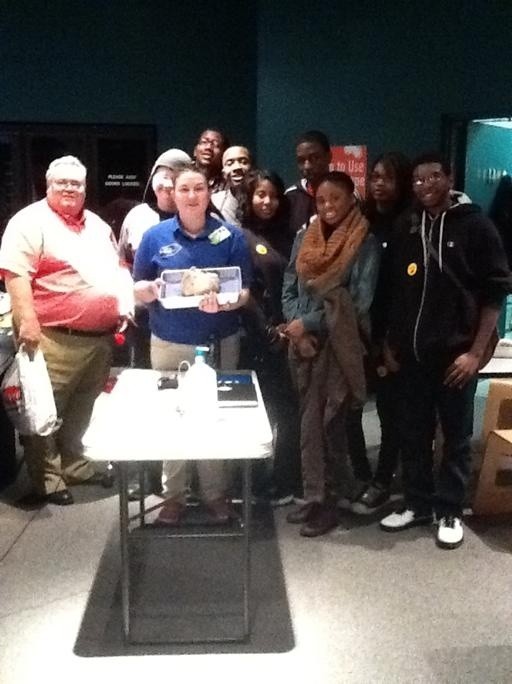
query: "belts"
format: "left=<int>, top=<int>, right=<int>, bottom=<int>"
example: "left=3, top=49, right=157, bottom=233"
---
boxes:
left=50, top=326, right=105, bottom=337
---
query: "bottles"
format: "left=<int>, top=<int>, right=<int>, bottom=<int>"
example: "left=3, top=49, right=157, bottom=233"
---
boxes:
left=102, top=334, right=126, bottom=394
left=502, top=293, right=512, bottom=341
left=179, top=345, right=221, bottom=434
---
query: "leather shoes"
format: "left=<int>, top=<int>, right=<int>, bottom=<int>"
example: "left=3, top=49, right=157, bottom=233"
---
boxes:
left=46, top=489, right=75, bottom=505
left=83, top=473, right=107, bottom=484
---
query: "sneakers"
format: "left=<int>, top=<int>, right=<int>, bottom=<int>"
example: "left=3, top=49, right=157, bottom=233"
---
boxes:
left=182, top=470, right=201, bottom=507
left=204, top=504, right=239, bottom=524
left=435, top=513, right=464, bottom=550
left=379, top=508, right=434, bottom=533
left=156, top=504, right=188, bottom=526
left=254, top=479, right=391, bottom=537
left=128, top=468, right=154, bottom=500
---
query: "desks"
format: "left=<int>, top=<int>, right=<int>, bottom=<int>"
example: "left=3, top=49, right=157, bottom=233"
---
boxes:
left=81, top=367, right=274, bottom=648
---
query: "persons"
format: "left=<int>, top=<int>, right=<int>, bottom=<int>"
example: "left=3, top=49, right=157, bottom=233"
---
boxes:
left=1, top=153, right=136, bottom=504
left=121, top=120, right=512, bottom=548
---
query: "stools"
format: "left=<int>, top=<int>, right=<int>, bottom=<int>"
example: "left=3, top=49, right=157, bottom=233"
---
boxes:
left=469, top=423, right=512, bottom=524
left=481, top=375, right=511, bottom=440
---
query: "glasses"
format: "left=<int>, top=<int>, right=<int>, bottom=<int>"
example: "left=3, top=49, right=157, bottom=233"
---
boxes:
left=368, top=170, right=392, bottom=183
left=411, top=172, right=443, bottom=187
